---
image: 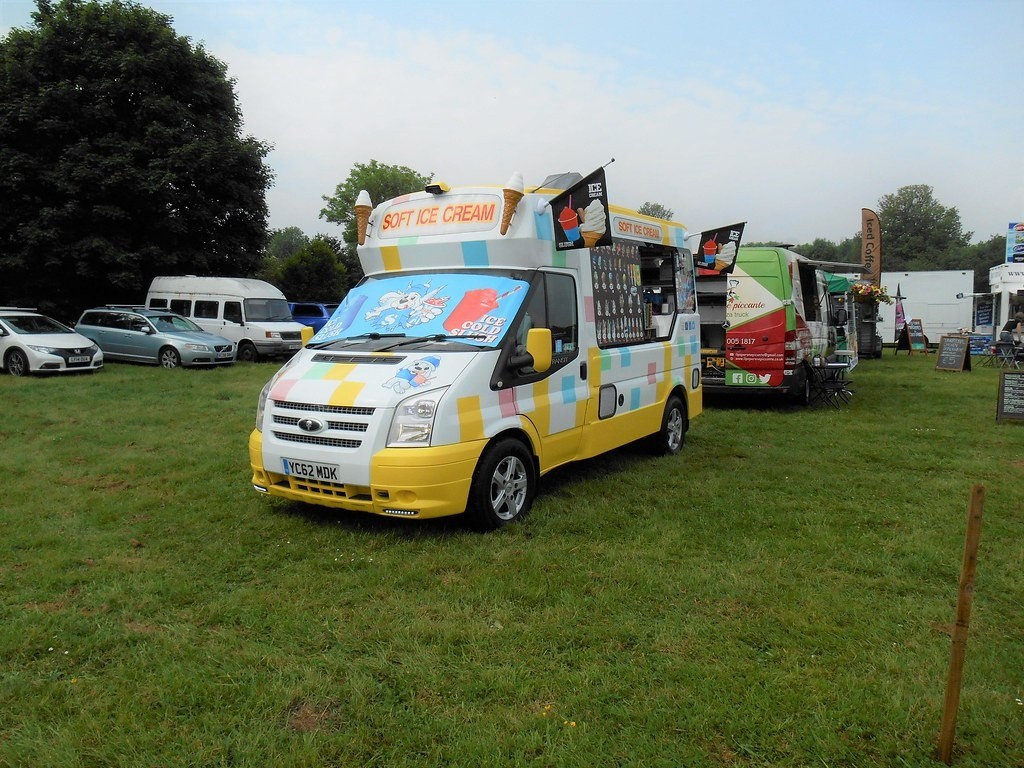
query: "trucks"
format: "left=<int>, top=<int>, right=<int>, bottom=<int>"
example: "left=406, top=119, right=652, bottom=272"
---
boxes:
left=697, top=246, right=873, bottom=404
left=249, top=181, right=702, bottom=531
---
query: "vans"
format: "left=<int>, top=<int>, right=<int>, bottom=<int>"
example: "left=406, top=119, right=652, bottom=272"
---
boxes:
left=145, top=274, right=309, bottom=362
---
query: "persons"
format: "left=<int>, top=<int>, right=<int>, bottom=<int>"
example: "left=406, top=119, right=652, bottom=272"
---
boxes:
left=999, top=312, right=1023, bottom=366
left=516, top=312, right=534, bottom=372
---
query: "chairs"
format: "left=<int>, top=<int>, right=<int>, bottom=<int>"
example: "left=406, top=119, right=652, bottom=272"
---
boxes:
left=973, top=341, right=1022, bottom=370
left=801, top=360, right=857, bottom=411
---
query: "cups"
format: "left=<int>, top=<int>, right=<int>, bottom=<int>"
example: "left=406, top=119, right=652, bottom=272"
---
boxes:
left=703, top=246, right=717, bottom=263
left=558, top=213, right=581, bottom=242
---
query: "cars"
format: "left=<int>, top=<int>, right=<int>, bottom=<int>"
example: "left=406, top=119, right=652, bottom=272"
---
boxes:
left=0, top=307, right=104, bottom=377
left=75, top=303, right=237, bottom=370
left=289, top=301, right=339, bottom=334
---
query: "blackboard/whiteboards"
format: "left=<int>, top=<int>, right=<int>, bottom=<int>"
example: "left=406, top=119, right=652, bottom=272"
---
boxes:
left=935, top=335, right=969, bottom=371
left=906, top=318, right=927, bottom=351
left=998, top=370, right=1024, bottom=420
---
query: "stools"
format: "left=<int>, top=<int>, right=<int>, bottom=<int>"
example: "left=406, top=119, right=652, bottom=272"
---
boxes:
left=833, top=350, right=855, bottom=385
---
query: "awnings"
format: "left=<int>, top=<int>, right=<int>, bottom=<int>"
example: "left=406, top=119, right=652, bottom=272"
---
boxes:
left=825, top=273, right=854, bottom=292
left=797, top=259, right=873, bottom=308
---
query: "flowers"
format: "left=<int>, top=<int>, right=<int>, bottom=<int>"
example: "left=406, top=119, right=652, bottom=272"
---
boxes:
left=848, top=283, right=894, bottom=305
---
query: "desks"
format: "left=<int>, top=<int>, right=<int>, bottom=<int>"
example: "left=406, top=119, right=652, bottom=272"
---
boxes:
left=988, top=341, right=1016, bottom=367
left=812, top=362, right=850, bottom=404
